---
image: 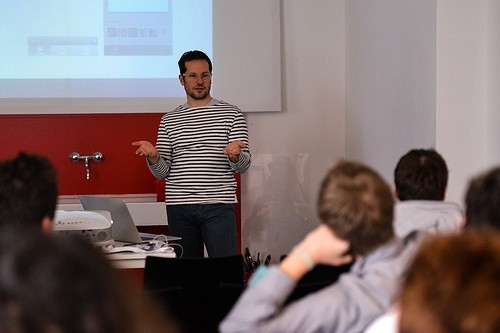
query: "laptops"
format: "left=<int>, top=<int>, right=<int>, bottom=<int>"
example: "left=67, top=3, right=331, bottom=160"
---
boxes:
left=78, top=196, right=182, bottom=243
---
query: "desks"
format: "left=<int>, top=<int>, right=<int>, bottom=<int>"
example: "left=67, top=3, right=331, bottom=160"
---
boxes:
left=106, top=251, right=178, bottom=333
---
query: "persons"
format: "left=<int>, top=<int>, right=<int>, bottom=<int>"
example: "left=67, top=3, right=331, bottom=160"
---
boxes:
left=130, top=49, right=252, bottom=258
left=218, top=160, right=430, bottom=333
left=389, top=147, right=500, bottom=333
left=0, top=151, right=159, bottom=333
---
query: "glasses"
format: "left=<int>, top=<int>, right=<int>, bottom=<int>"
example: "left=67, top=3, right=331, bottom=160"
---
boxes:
left=183, top=73, right=213, bottom=81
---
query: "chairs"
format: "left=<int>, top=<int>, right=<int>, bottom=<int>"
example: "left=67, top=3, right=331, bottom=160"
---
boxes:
left=142, top=254, right=246, bottom=333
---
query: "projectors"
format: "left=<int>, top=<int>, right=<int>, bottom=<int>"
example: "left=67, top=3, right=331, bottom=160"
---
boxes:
left=53, top=209, right=113, bottom=243
